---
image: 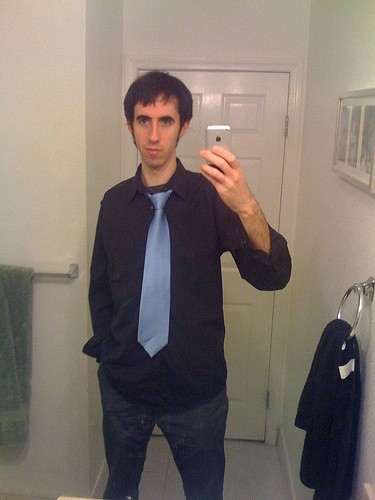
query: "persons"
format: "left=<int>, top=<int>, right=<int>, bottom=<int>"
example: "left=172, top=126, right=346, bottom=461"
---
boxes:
left=80, top=73, right=293, bottom=500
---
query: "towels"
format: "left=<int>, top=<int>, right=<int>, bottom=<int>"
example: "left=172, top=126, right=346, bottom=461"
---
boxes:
left=294, top=318, right=362, bottom=500
left=0, top=263, right=35, bottom=467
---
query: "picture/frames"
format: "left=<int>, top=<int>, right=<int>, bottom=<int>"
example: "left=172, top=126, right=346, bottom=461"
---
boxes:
left=332, top=88, right=375, bottom=194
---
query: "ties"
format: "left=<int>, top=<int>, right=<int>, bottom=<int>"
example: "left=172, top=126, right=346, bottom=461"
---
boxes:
left=138, top=189, right=170, bottom=358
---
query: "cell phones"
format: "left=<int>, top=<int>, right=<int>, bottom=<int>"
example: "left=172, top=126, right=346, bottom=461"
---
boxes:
left=206, top=125, right=231, bottom=166
